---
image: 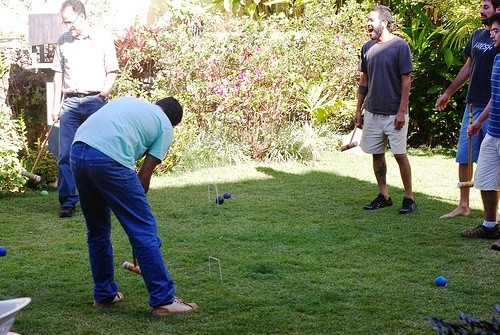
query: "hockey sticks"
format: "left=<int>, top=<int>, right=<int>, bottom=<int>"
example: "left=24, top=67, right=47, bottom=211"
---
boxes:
left=456, top=103, right=475, bottom=188
left=20, top=117, right=59, bottom=181
left=340, top=104, right=365, bottom=152
left=123, top=244, right=143, bottom=275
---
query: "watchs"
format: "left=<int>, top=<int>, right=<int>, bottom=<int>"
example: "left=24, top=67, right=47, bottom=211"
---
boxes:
left=98, top=94, right=108, bottom=102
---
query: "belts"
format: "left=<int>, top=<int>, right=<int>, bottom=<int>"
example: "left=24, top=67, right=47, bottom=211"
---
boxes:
left=63, top=91, right=101, bottom=98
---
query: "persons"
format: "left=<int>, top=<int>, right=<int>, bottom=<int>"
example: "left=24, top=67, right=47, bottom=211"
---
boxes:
left=435, top=0, right=500, bottom=219
left=462, top=12, right=500, bottom=251
left=52, top=0, right=129, bottom=217
left=354, top=6, right=417, bottom=215
left=70, top=96, right=197, bottom=316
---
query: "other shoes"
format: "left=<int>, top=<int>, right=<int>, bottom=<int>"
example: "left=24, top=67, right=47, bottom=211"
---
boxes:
left=60, top=207, right=75, bottom=217
left=93, top=292, right=124, bottom=308
left=152, top=297, right=197, bottom=317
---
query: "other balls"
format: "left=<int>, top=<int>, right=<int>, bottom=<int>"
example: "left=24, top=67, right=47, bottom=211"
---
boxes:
left=223, top=193, right=232, bottom=199
left=0, top=247, right=8, bottom=257
left=433, top=274, right=449, bottom=288
left=215, top=196, right=225, bottom=205
left=37, top=189, right=51, bottom=197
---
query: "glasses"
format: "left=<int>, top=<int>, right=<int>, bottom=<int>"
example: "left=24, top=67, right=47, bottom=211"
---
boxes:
left=489, top=30, right=500, bottom=35
left=61, top=14, right=80, bottom=26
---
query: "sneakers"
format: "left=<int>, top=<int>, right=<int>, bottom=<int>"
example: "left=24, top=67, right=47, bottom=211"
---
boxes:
left=492, top=239, right=500, bottom=251
left=399, top=196, right=417, bottom=214
left=363, top=193, right=393, bottom=210
left=461, top=223, right=500, bottom=240
